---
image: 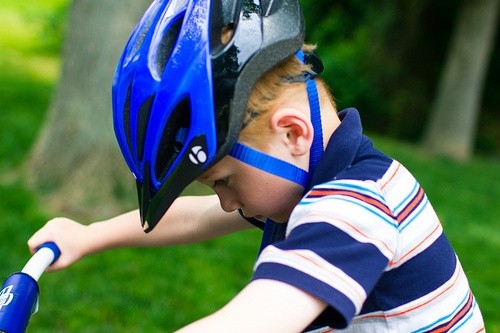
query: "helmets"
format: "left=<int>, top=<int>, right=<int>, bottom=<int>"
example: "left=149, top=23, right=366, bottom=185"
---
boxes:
left=111, top=0, right=306, bottom=233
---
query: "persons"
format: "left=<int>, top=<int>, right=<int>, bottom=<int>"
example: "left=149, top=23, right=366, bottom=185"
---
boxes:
left=26, top=1, right=488, bottom=333
